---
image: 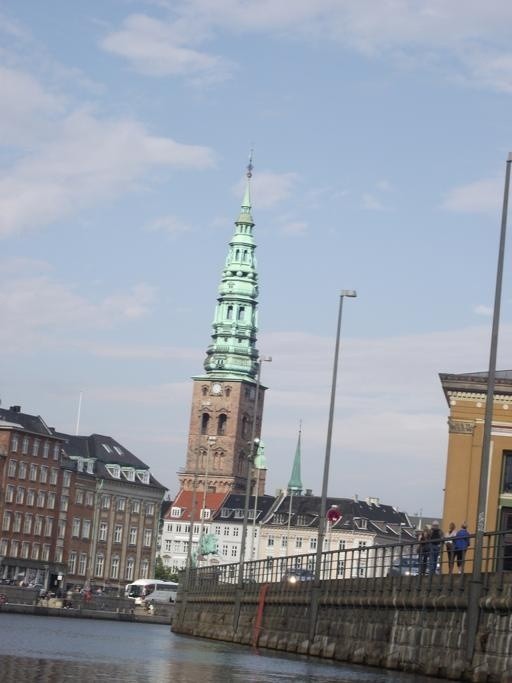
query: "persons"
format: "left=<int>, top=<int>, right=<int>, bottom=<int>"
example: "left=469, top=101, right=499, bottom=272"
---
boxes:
left=426, top=520, right=445, bottom=574
left=445, top=522, right=457, bottom=574
left=456, top=521, right=470, bottom=574
left=414, top=531, right=430, bottom=575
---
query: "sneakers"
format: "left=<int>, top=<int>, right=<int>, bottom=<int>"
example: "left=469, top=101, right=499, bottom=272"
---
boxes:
left=447, top=565, right=463, bottom=575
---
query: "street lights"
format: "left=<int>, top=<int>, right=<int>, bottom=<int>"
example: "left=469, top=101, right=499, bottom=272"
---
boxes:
left=57, top=572, right=65, bottom=598
left=315, top=289, right=357, bottom=586
left=238, top=356, right=272, bottom=588
left=285, top=486, right=302, bottom=565
left=195, top=436, right=217, bottom=590
left=399, top=522, right=406, bottom=543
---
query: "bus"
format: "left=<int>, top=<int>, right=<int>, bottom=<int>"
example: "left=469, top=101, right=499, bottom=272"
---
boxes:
left=125, top=578, right=179, bottom=606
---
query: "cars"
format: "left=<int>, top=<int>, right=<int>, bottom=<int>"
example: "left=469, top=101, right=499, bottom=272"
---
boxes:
left=280, top=568, right=315, bottom=584
left=387, top=555, right=441, bottom=576
